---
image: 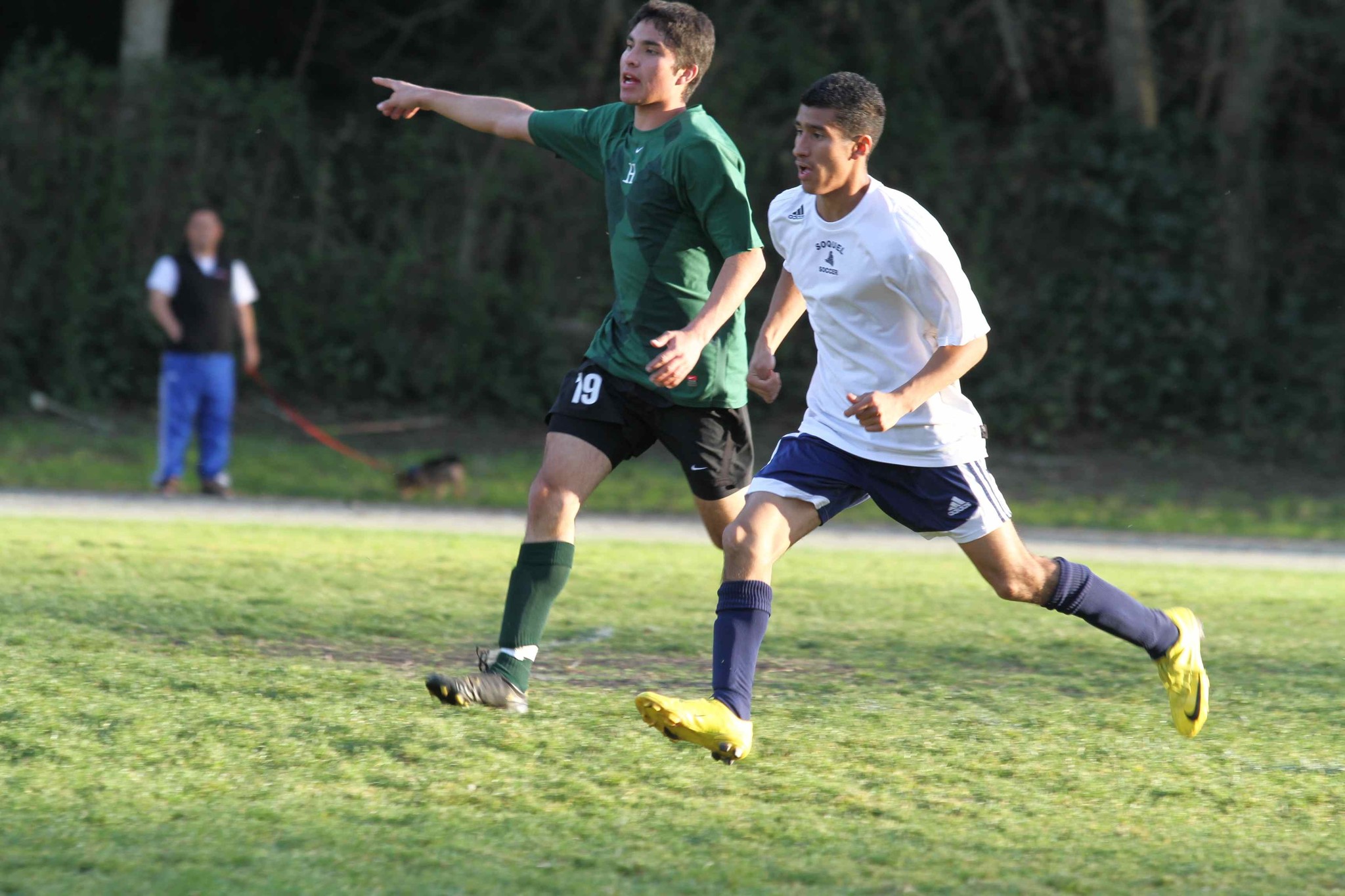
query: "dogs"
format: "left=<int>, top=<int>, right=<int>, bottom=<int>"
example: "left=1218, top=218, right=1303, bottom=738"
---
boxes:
left=393, top=453, right=466, bottom=500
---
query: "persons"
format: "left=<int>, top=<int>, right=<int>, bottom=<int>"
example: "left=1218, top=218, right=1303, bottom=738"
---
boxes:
left=368, top=2, right=766, bottom=717
left=633, top=67, right=1212, bottom=763
left=144, top=206, right=262, bottom=501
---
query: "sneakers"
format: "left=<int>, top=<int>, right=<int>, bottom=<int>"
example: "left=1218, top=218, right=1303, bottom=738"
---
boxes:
left=156, top=476, right=182, bottom=493
left=429, top=669, right=528, bottom=713
left=635, top=693, right=754, bottom=762
left=1152, top=609, right=1209, bottom=738
left=203, top=476, right=232, bottom=499
